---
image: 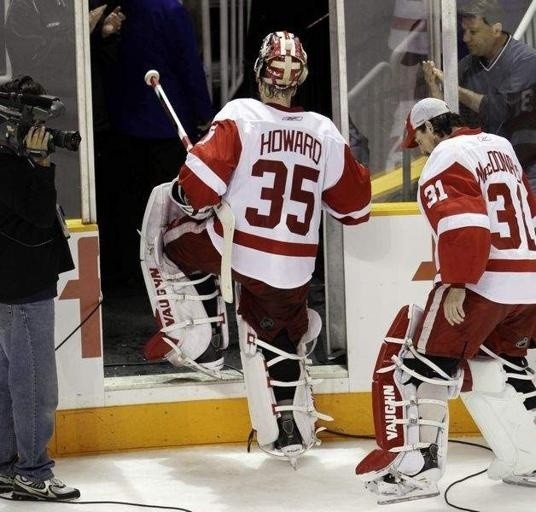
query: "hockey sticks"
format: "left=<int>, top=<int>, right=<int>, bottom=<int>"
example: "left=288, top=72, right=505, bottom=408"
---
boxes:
left=144, top=68, right=235, bottom=306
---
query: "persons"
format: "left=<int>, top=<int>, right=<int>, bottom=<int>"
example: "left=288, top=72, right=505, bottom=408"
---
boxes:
left=344, top=0, right=536, bottom=201
left=355, top=96, right=536, bottom=493
left=137, top=30, right=371, bottom=471
left=0, top=76, right=83, bottom=502
left=1, top=0, right=329, bottom=291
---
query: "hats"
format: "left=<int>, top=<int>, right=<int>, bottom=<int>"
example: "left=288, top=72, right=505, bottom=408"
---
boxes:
left=400, top=97, right=453, bottom=149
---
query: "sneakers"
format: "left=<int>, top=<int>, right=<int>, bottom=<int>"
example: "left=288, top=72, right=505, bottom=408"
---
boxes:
left=272, top=416, right=303, bottom=453
left=189, top=330, right=225, bottom=372
left=0, top=473, right=15, bottom=500
left=365, top=443, right=442, bottom=494
left=11, top=473, right=81, bottom=502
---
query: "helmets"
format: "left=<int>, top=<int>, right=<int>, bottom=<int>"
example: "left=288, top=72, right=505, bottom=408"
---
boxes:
left=253, top=29, right=309, bottom=91
